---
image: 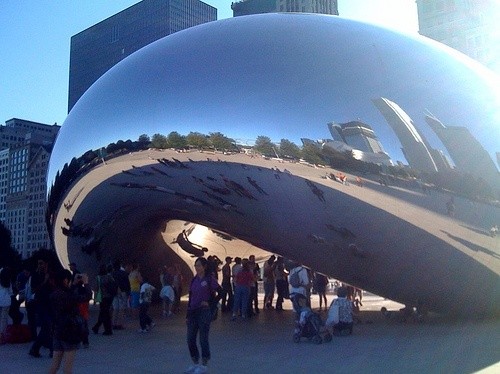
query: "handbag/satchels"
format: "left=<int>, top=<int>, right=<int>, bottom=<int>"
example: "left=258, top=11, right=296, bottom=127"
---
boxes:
left=325, top=299, right=339, bottom=327
left=61, top=313, right=89, bottom=344
left=138, top=289, right=149, bottom=304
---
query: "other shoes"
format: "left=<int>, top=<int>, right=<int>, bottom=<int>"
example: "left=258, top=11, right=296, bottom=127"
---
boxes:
left=136, top=324, right=153, bottom=333
left=333, top=328, right=350, bottom=336
left=113, top=325, right=126, bottom=330
left=91, top=326, right=113, bottom=335
left=184, top=363, right=208, bottom=374
left=222, top=303, right=288, bottom=321
left=28, top=350, right=43, bottom=358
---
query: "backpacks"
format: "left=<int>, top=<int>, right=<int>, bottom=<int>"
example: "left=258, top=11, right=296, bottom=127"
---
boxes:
left=99, top=274, right=116, bottom=298
left=192, top=276, right=219, bottom=321
left=289, top=268, right=306, bottom=288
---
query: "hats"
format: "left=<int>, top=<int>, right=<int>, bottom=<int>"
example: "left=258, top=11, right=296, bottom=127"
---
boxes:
left=226, top=257, right=233, bottom=262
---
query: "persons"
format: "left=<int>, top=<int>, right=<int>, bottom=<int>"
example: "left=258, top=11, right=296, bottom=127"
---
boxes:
left=58, top=148, right=326, bottom=254
left=0, top=251, right=95, bottom=374
left=324, top=169, right=390, bottom=198
left=91, top=261, right=182, bottom=337
left=206, top=254, right=262, bottom=321
left=443, top=195, right=457, bottom=218
left=183, top=256, right=226, bottom=374
left=311, top=222, right=367, bottom=258
left=261, top=254, right=365, bottom=341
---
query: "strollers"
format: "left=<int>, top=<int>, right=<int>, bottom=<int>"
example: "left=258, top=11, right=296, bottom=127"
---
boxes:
left=286, top=292, right=335, bottom=344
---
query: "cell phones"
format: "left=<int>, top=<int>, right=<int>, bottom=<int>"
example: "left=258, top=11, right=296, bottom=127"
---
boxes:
left=76, top=274, right=83, bottom=280
